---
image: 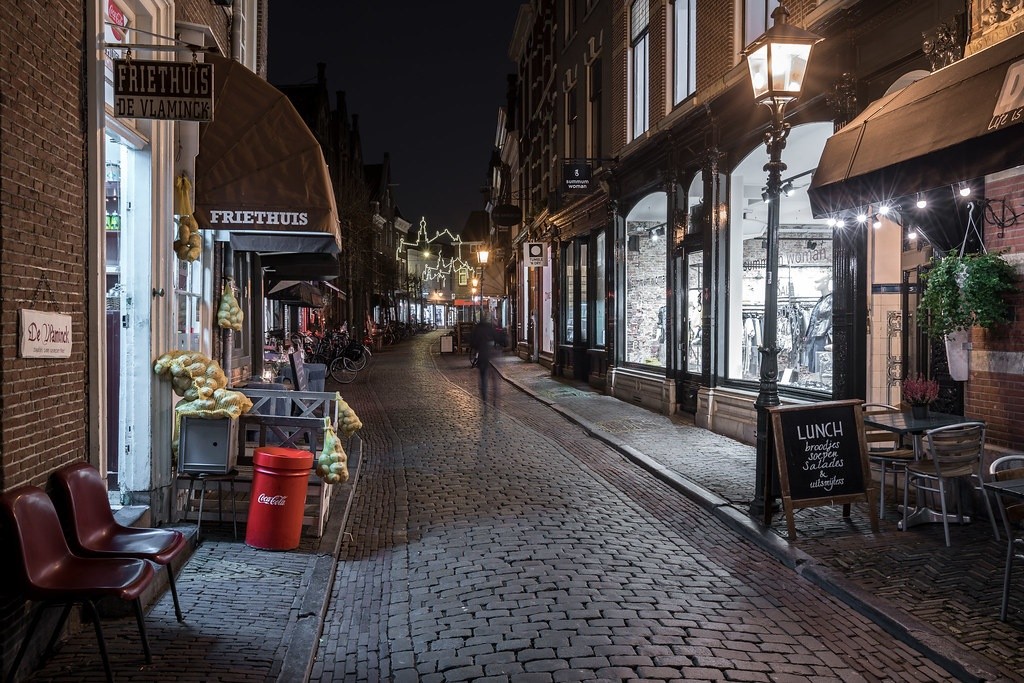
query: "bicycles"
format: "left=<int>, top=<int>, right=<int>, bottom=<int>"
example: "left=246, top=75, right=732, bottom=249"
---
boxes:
left=290, top=330, right=372, bottom=385
left=470, top=346, right=479, bottom=368
left=341, top=318, right=438, bottom=353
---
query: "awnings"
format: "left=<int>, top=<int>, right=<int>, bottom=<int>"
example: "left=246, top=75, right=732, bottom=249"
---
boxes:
left=198, top=55, right=343, bottom=307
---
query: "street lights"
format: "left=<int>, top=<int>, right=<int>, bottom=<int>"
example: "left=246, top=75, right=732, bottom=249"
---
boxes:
left=475, top=239, right=490, bottom=322
left=432, top=293, right=439, bottom=325
left=741, top=6, right=826, bottom=514
left=472, top=275, right=479, bottom=322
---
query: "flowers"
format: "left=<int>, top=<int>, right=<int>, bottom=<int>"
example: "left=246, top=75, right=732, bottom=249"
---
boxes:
left=901, top=372, right=939, bottom=402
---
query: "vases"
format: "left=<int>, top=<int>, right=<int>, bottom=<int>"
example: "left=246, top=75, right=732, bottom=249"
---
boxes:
left=911, top=404, right=929, bottom=419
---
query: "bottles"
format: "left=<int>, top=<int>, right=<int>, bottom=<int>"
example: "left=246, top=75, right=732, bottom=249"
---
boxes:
left=106, top=210, right=118, bottom=229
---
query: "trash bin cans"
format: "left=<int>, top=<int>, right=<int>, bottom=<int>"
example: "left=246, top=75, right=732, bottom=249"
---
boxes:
left=440, top=335, right=454, bottom=353
left=244, top=447, right=314, bottom=551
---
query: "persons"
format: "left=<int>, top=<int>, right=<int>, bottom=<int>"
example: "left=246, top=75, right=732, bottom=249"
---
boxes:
left=472, top=315, right=502, bottom=378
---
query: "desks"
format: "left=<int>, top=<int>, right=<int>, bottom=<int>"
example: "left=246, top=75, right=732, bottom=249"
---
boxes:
left=983, top=480, right=1024, bottom=502
left=863, top=411, right=985, bottom=527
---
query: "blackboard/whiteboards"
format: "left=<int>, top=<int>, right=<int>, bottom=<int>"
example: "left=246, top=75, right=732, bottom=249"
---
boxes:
left=765, top=399, right=877, bottom=510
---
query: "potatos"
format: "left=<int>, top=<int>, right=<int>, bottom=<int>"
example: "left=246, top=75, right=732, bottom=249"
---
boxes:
left=315, top=439, right=349, bottom=484
left=174, top=216, right=200, bottom=261
left=337, top=401, right=362, bottom=438
left=217, top=294, right=244, bottom=331
left=154, top=352, right=253, bottom=417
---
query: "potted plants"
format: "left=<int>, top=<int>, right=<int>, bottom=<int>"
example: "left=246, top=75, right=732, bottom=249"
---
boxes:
left=917, top=247, right=1024, bottom=341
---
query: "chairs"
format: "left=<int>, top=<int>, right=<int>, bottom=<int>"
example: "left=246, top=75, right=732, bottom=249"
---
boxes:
left=862, top=403, right=1024, bottom=620
left=0, top=462, right=186, bottom=683
